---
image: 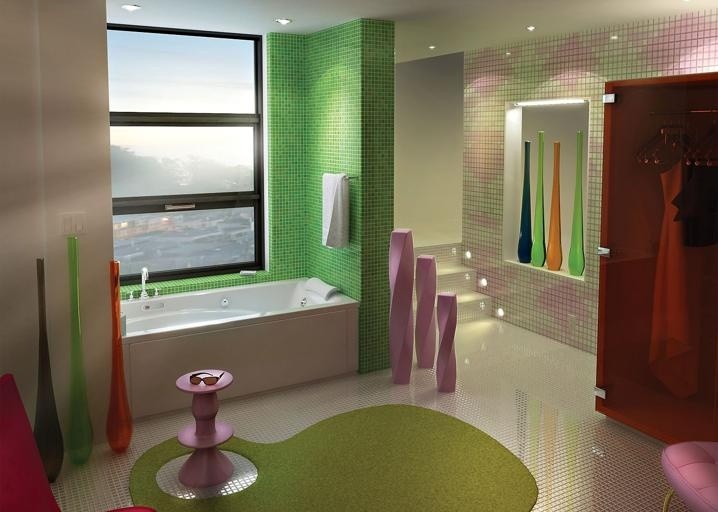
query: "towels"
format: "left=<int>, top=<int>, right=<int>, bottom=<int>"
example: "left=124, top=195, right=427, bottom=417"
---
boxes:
left=321, top=173, right=350, bottom=254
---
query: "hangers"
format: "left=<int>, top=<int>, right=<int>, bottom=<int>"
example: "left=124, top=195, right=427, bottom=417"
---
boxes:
left=630, top=123, right=718, bottom=171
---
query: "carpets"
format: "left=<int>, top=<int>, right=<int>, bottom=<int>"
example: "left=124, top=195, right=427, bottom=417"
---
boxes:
left=127, top=400, right=540, bottom=512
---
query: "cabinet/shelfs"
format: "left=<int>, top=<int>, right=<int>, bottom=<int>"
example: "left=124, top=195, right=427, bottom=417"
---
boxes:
left=174, top=369, right=237, bottom=487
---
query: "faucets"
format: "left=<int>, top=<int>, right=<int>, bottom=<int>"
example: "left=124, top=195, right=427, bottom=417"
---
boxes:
left=140, top=267, right=150, bottom=300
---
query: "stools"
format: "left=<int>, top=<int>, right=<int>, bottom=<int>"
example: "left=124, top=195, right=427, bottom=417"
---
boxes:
left=660, top=438, right=718, bottom=510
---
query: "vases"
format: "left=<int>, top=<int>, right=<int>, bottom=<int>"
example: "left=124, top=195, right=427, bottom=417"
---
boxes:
left=517, top=128, right=589, bottom=280
left=30, top=235, right=134, bottom=486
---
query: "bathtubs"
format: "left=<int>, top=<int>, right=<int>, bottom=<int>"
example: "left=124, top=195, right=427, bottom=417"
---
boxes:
left=119, top=276, right=360, bottom=342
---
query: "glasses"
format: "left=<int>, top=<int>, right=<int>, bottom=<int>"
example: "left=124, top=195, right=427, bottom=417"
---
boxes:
left=189, top=371, right=225, bottom=385
